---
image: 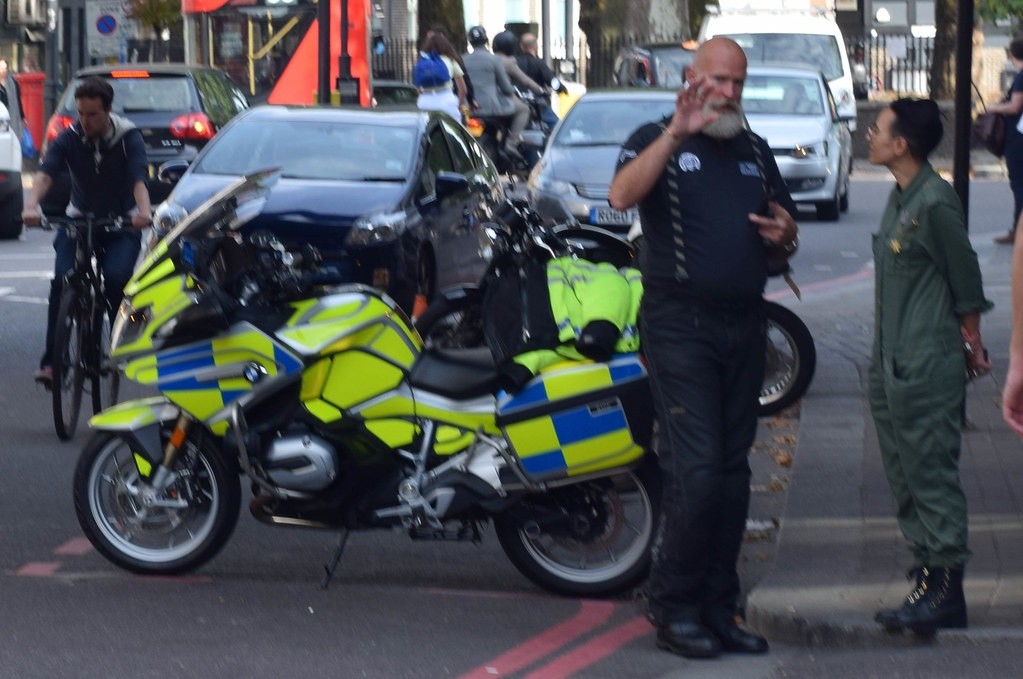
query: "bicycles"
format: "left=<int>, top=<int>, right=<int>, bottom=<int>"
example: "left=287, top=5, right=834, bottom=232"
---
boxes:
left=38, top=207, right=152, bottom=444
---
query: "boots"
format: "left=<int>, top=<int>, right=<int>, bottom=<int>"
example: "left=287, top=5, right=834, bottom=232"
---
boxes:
left=874, top=565, right=943, bottom=626
left=893, top=568, right=967, bottom=627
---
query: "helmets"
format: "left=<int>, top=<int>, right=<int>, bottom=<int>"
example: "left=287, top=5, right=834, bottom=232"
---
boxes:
left=494, top=31, right=517, bottom=55
left=468, top=27, right=487, bottom=46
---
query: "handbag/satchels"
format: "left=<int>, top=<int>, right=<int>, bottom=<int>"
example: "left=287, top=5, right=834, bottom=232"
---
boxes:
left=975, top=113, right=1006, bottom=158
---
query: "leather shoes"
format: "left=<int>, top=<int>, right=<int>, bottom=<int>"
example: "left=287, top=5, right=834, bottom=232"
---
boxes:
left=699, top=608, right=769, bottom=654
left=657, top=609, right=720, bottom=657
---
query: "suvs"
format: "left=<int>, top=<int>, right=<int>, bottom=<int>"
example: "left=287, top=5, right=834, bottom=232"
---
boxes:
left=609, top=40, right=705, bottom=90
left=38, top=60, right=258, bottom=201
left=358, top=82, right=426, bottom=107
left=0, top=99, right=27, bottom=240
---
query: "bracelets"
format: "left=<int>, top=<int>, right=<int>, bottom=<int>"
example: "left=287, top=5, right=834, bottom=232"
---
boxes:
left=961, top=326, right=980, bottom=350
left=665, top=126, right=680, bottom=141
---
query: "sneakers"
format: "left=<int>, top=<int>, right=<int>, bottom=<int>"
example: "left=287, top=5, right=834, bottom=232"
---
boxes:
left=35, top=365, right=53, bottom=386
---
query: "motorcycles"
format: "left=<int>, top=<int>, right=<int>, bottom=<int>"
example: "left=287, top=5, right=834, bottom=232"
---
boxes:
left=463, top=77, right=564, bottom=181
left=472, top=172, right=817, bottom=415
left=70, top=166, right=662, bottom=599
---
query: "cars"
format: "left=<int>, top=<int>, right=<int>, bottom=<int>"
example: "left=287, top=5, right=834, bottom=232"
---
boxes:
left=142, top=103, right=509, bottom=329
left=732, top=64, right=855, bottom=223
left=516, top=88, right=679, bottom=244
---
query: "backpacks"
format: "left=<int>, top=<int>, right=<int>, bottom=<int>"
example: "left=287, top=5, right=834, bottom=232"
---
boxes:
left=412, top=50, right=450, bottom=87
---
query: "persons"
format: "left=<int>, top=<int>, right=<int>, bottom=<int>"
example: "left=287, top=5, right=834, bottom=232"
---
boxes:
left=865, top=98, right=992, bottom=633
left=987, top=37, right=1023, bottom=244
left=21, top=78, right=151, bottom=383
left=0, top=59, right=27, bottom=124
left=609, top=36, right=799, bottom=660
left=413, top=23, right=568, bottom=167
left=1003, top=210, right=1022, bottom=433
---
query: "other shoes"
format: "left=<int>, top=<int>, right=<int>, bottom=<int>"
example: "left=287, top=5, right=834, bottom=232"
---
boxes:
left=994, top=230, right=1014, bottom=244
left=506, top=144, right=524, bottom=161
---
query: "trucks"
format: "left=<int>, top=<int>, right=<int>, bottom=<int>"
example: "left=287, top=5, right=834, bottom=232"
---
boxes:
left=695, top=6, right=865, bottom=172
